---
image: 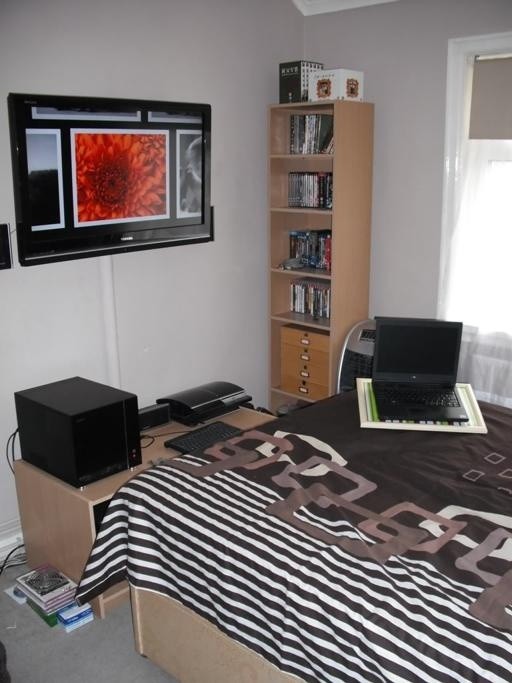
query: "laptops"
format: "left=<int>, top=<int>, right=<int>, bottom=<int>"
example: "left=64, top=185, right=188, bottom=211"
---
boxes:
left=372, top=318, right=469, bottom=422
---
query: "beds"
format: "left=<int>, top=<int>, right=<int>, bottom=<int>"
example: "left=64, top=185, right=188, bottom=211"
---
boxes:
left=130, top=388, right=512, bottom=682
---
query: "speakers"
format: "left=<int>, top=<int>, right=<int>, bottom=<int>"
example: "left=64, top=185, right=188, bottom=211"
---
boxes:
left=14, top=375, right=142, bottom=487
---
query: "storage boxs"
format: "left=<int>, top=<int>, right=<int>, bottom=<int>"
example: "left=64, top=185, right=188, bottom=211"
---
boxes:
left=308, top=68, right=364, bottom=100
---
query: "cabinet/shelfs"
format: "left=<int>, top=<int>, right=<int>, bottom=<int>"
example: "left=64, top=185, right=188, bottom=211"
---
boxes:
left=12, top=404, right=276, bottom=621
left=269, top=100, right=374, bottom=416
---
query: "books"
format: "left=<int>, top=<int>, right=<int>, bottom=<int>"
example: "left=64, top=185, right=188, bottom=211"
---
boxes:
left=288, top=171, right=333, bottom=211
left=289, top=278, right=330, bottom=320
left=289, top=230, right=333, bottom=273
left=287, top=113, right=335, bottom=154
left=15, top=562, right=95, bottom=634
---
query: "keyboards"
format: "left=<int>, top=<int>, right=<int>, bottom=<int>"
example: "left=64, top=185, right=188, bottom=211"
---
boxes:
left=164, top=420, right=242, bottom=454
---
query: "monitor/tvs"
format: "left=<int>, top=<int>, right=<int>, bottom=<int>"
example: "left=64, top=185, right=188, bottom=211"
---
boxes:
left=7, top=92, right=214, bottom=267
